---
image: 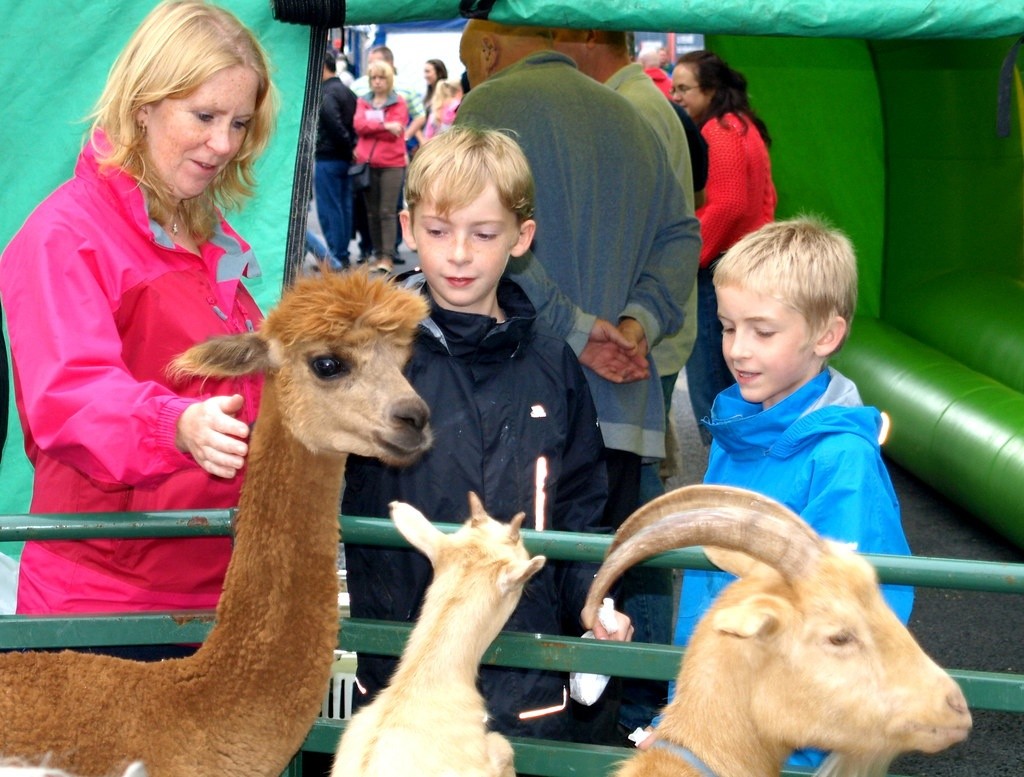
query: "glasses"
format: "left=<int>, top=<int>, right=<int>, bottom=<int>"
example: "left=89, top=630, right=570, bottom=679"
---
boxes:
left=668, top=84, right=703, bottom=96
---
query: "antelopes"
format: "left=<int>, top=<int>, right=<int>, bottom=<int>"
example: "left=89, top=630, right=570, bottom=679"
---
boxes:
left=582, top=487, right=972, bottom=777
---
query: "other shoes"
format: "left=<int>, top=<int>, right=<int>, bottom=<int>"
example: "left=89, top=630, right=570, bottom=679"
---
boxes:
left=368, top=266, right=378, bottom=273
left=378, top=264, right=391, bottom=274
left=393, top=254, right=405, bottom=264
left=340, top=257, right=350, bottom=269
left=356, top=256, right=368, bottom=264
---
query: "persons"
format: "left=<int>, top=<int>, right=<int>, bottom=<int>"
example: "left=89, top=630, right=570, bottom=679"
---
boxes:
left=314, top=41, right=471, bottom=271
left=550, top=27, right=699, bottom=485
left=639, top=45, right=776, bottom=441
left=642, top=218, right=915, bottom=766
left=340, top=130, right=632, bottom=744
left=0, top=0, right=275, bottom=656
left=460, top=18, right=702, bottom=745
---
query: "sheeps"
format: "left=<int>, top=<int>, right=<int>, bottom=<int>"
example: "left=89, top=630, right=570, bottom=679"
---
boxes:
left=331, top=491, right=549, bottom=776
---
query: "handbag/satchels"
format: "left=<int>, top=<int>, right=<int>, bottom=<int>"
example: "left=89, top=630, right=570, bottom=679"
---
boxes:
left=347, top=162, right=370, bottom=189
